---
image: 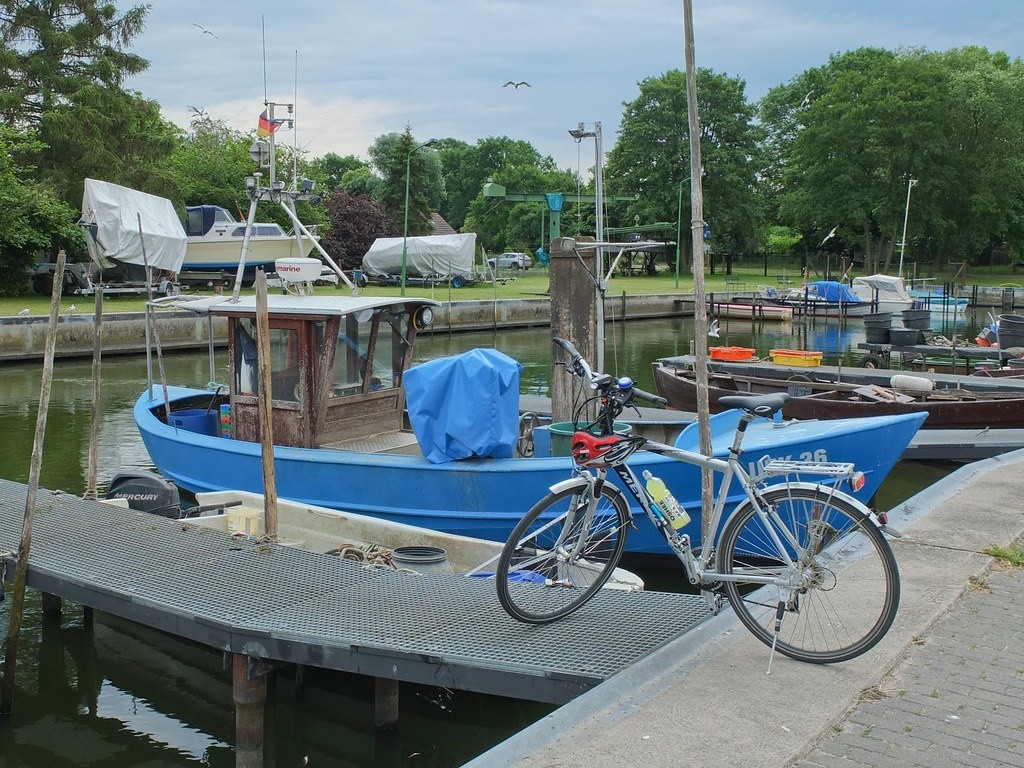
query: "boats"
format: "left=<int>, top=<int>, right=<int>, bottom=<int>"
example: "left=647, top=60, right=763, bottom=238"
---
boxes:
left=97, top=471, right=643, bottom=594
left=77, top=178, right=324, bottom=276
left=652, top=362, right=1024, bottom=429
left=135, top=14, right=929, bottom=573
left=362, top=233, right=477, bottom=275
left=706, top=274, right=969, bottom=321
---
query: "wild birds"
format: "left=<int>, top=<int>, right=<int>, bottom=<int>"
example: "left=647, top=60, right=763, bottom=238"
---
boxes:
left=709, top=319, right=720, bottom=339
left=18, top=308, right=30, bottom=316
left=193, top=23, right=217, bottom=39
left=502, top=81, right=532, bottom=90
left=64, top=304, right=76, bottom=315
left=800, top=90, right=813, bottom=107
left=820, top=226, right=838, bottom=246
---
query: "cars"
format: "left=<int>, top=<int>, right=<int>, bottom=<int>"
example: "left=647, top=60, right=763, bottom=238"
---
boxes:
left=485, top=253, right=532, bottom=271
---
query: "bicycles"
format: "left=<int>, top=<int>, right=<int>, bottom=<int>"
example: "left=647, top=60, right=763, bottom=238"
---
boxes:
left=496, top=336, right=902, bottom=675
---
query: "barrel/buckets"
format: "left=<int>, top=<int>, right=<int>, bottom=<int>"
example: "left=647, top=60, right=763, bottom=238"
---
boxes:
left=547, top=420, right=633, bottom=457
left=863, top=309, right=933, bottom=346
left=389, top=545, right=454, bottom=575
left=167, top=409, right=219, bottom=437
left=997, top=314, right=1024, bottom=366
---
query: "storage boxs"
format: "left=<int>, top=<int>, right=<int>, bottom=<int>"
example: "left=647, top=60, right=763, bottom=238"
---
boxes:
left=710, top=346, right=757, bottom=361
left=769, top=349, right=824, bottom=366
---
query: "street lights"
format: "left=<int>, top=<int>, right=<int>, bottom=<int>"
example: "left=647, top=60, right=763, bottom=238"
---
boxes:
left=400, top=139, right=438, bottom=295
left=675, top=173, right=706, bottom=289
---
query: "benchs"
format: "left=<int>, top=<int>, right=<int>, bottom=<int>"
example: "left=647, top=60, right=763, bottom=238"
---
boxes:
left=726, top=275, right=746, bottom=291
left=777, top=275, right=797, bottom=289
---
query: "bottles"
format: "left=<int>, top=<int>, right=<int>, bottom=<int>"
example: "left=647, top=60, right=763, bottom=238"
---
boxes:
left=642, top=470, right=692, bottom=530
